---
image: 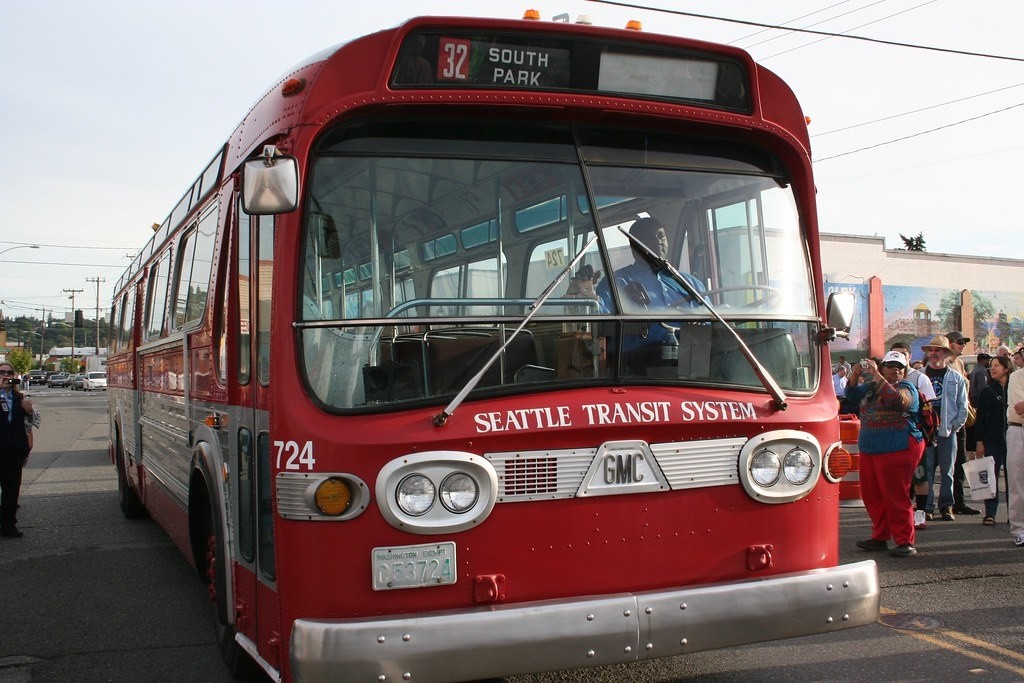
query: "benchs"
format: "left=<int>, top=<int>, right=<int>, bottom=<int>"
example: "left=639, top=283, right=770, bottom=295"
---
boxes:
left=371, top=322, right=563, bottom=399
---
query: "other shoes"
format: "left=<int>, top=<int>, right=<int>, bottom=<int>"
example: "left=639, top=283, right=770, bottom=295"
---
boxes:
left=1014, top=535, right=1024, bottom=545
left=856, top=539, right=887, bottom=551
left=942, top=506, right=955, bottom=521
left=953, top=504, right=980, bottom=514
left=926, top=512, right=934, bottom=520
left=0, top=531, right=23, bottom=538
left=888, top=544, right=917, bottom=556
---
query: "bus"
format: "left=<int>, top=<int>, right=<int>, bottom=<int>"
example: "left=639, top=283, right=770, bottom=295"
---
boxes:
left=108, top=10, right=881, bottom=683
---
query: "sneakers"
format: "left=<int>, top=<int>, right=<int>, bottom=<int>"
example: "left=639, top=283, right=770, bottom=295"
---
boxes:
left=914, top=510, right=926, bottom=528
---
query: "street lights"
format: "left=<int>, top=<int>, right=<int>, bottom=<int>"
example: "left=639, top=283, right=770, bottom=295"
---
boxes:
left=83, top=317, right=99, bottom=355
left=24, top=331, right=43, bottom=368
left=52, top=322, right=75, bottom=374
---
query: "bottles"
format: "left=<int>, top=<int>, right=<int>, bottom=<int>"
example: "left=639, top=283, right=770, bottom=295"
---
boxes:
left=661, top=327, right=680, bottom=383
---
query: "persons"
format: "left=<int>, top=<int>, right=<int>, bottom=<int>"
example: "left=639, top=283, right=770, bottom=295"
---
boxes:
left=13, top=384, right=34, bottom=509
left=1006, top=367, right=1024, bottom=545
left=23, top=373, right=30, bottom=391
left=18, top=371, right=22, bottom=384
left=0, top=362, right=33, bottom=537
left=596, top=217, right=716, bottom=381
left=830, top=330, right=1024, bottom=530
left=844, top=351, right=925, bottom=558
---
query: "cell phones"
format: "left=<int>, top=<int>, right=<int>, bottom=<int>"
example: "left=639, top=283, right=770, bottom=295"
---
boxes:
left=8, top=379, right=20, bottom=384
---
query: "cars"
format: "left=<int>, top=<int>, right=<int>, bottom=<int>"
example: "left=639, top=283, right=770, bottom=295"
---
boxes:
left=59, top=372, right=85, bottom=390
left=47, top=375, right=69, bottom=388
left=43, top=371, right=58, bottom=382
left=83, top=371, right=107, bottom=391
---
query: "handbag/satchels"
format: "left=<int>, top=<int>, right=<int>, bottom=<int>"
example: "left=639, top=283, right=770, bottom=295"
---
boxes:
left=961, top=455, right=996, bottom=500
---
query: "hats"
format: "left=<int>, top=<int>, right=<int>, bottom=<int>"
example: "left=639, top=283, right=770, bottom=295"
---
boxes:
left=881, top=351, right=907, bottom=366
left=921, top=335, right=955, bottom=355
left=977, top=353, right=993, bottom=359
left=1019, top=348, right=1024, bottom=352
left=945, top=332, right=970, bottom=342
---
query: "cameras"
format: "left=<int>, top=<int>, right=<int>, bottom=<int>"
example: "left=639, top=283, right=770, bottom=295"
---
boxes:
left=860, top=360, right=869, bottom=368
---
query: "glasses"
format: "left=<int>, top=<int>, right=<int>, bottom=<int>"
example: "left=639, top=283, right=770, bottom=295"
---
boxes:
left=956, top=342, right=966, bottom=345
left=1003, top=353, right=1011, bottom=357
left=928, top=348, right=942, bottom=352
left=884, top=362, right=905, bottom=370
left=0, top=370, right=13, bottom=375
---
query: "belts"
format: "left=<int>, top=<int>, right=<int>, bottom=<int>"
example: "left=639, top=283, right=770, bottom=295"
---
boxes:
left=1010, top=422, right=1024, bottom=427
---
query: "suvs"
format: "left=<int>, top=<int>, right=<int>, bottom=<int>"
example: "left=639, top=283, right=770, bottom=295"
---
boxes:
left=28, top=369, right=46, bottom=386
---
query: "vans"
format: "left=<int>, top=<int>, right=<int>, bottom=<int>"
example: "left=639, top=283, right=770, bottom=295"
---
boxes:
left=958, top=355, right=996, bottom=373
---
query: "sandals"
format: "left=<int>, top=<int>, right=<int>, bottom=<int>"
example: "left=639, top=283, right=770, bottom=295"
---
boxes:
left=983, top=515, right=995, bottom=525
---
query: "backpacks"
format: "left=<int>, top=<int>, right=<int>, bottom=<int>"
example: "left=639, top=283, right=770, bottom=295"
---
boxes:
left=863, top=380, right=940, bottom=447
